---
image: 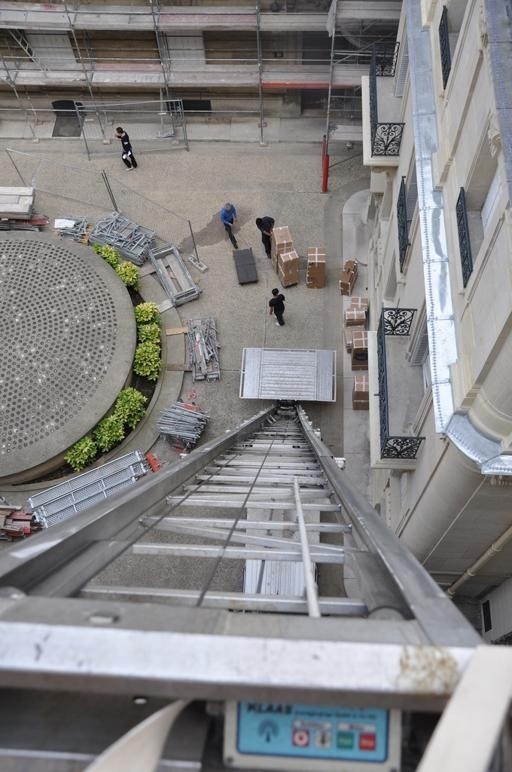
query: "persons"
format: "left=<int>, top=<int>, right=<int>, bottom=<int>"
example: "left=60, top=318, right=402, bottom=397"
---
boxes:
left=221, top=202, right=239, bottom=251
left=114, top=126, right=138, bottom=171
left=269, top=289, right=286, bottom=328
left=254, top=216, right=276, bottom=260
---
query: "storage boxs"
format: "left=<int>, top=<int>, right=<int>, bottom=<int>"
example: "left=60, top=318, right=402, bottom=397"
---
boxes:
left=271, top=226, right=370, bottom=411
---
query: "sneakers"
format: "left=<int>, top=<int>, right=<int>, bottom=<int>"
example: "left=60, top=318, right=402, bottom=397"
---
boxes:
left=234, top=245, right=238, bottom=249
left=267, top=254, right=271, bottom=258
left=126, top=165, right=133, bottom=171
left=275, top=316, right=278, bottom=318
left=276, top=322, right=281, bottom=327
